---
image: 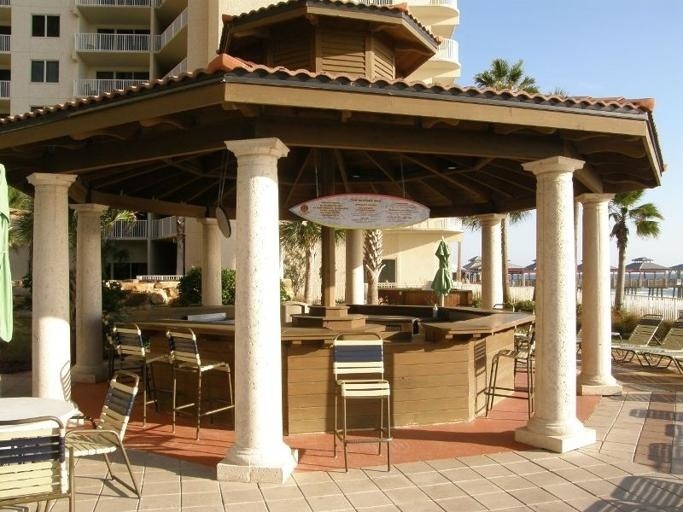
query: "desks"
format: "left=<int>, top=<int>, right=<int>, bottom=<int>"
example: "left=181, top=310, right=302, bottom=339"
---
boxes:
left=2, top=395, right=87, bottom=492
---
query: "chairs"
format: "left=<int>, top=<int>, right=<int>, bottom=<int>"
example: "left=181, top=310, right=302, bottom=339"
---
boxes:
left=332, top=330, right=393, bottom=474
left=1, top=415, right=74, bottom=512
left=577, top=314, right=683, bottom=377
left=62, top=370, right=141, bottom=512
left=166, top=323, right=234, bottom=441
left=485, top=320, right=536, bottom=422
left=108, top=318, right=174, bottom=417
